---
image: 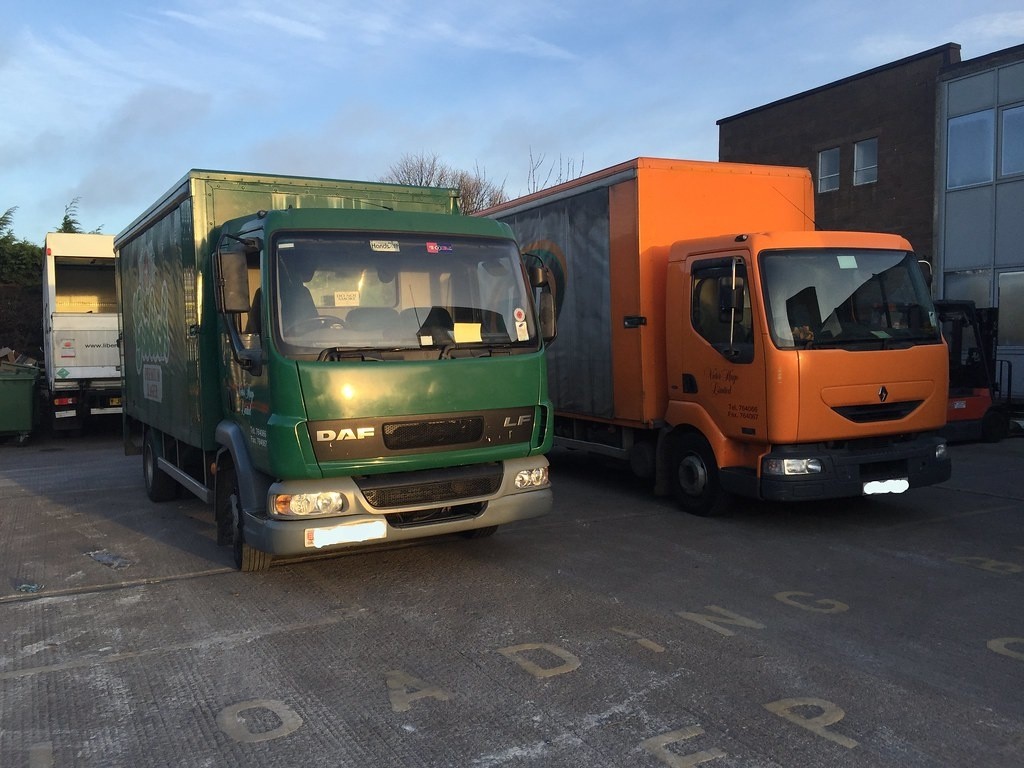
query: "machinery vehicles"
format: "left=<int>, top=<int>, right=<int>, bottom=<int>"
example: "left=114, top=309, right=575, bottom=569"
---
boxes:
left=898, top=300, right=1023, bottom=445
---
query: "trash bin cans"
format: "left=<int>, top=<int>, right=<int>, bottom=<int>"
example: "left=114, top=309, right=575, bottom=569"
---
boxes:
left=0, top=367, right=40, bottom=446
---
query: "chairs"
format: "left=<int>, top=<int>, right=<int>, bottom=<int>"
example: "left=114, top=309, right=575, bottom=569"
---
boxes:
left=250, top=261, right=321, bottom=336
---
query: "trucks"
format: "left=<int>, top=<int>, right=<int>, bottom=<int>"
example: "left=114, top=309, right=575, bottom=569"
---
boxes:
left=453, top=156, right=953, bottom=519
left=114, top=168, right=559, bottom=574
left=39, top=231, right=123, bottom=439
